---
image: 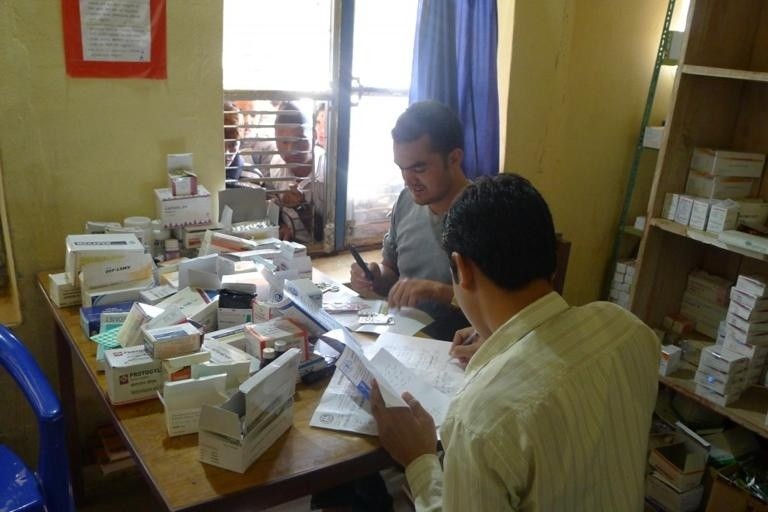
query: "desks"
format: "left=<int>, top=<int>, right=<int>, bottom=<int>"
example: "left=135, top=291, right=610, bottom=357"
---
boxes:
left=32, top=267, right=440, bottom=510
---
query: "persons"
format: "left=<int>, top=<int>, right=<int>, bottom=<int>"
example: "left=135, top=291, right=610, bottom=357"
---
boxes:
left=351, top=101, right=474, bottom=307
left=370, top=176, right=660, bottom=512
left=225, top=101, right=266, bottom=188
left=270, top=103, right=328, bottom=239
left=310, top=105, right=332, bottom=153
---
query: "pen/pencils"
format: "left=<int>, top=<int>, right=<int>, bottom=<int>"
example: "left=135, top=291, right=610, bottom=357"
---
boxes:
left=350, top=246, right=374, bottom=281
left=444, top=330, right=477, bottom=363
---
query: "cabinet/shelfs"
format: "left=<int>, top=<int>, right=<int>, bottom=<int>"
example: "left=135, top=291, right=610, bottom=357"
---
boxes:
left=626, top=0, right=768, bottom=512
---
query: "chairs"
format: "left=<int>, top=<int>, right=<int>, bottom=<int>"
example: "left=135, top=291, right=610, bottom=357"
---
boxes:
left=0, top=324, right=75, bottom=512
